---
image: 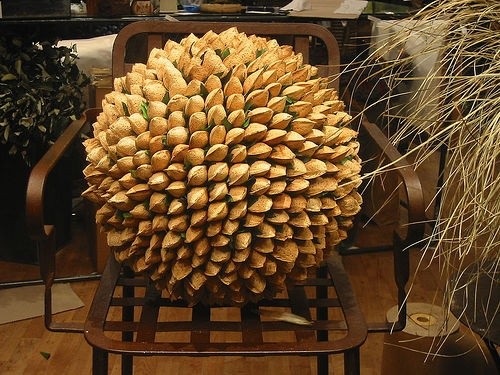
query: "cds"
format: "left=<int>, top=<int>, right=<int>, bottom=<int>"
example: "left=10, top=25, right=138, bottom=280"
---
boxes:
left=386, top=302, right=460, bottom=338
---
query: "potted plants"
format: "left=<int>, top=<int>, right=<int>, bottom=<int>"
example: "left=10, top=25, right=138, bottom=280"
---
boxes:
left=0, top=35, right=92, bottom=265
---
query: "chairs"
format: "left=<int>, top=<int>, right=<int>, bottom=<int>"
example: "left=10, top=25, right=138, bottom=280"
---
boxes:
left=25, top=19, right=426, bottom=375
left=333, top=18, right=461, bottom=255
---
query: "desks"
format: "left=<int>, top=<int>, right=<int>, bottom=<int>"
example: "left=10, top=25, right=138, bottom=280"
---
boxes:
left=121, top=1, right=422, bottom=94
left=448, top=260, right=500, bottom=375
